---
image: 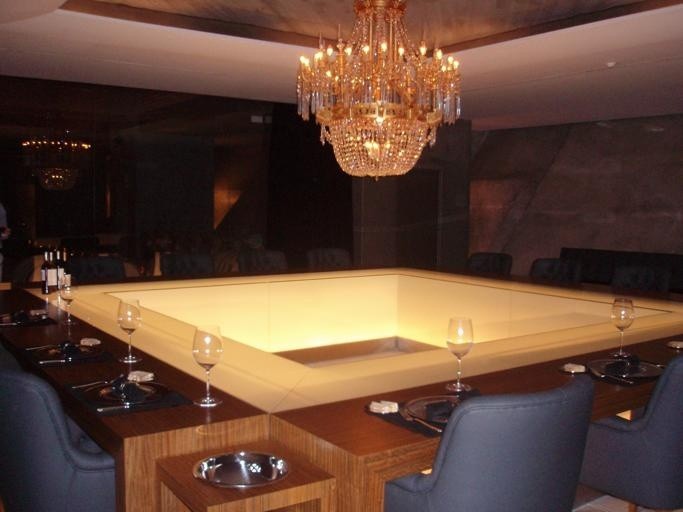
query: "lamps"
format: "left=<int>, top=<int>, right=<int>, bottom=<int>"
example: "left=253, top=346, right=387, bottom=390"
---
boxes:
left=19, top=113, right=96, bottom=191
left=296, top=0, right=463, bottom=180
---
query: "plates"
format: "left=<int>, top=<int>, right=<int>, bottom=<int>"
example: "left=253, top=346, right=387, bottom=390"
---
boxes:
left=190, top=450, right=289, bottom=490
left=591, top=357, right=665, bottom=377
left=406, top=396, right=463, bottom=422
left=85, top=383, right=170, bottom=404
left=0, top=309, right=104, bottom=360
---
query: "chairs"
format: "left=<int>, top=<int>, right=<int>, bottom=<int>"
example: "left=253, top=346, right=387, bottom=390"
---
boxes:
left=1, top=346, right=118, bottom=511
left=578, top=356, right=682, bottom=511
left=384, top=374, right=594, bottom=512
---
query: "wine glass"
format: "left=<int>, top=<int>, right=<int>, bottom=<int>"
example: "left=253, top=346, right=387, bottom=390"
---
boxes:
left=192, top=326, right=224, bottom=407
left=58, top=278, right=78, bottom=326
left=118, top=298, right=143, bottom=363
left=445, top=317, right=475, bottom=392
left=610, top=296, right=634, bottom=359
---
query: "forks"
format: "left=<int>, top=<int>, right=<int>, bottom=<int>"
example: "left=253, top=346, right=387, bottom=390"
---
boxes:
left=589, top=368, right=631, bottom=386
left=70, top=373, right=123, bottom=392
left=397, top=408, right=442, bottom=434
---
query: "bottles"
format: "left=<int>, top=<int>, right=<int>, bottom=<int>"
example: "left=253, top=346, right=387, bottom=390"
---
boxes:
left=39, top=247, right=74, bottom=293
